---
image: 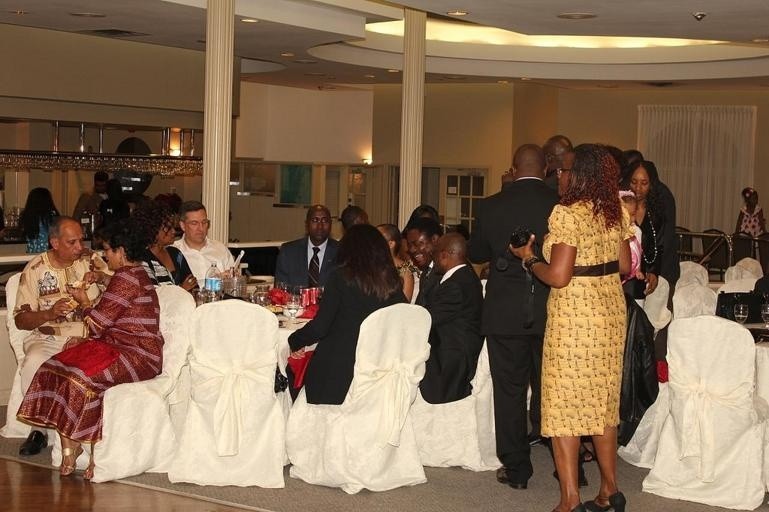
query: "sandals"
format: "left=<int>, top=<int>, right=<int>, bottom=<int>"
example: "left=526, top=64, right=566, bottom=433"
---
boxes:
left=58, top=440, right=83, bottom=476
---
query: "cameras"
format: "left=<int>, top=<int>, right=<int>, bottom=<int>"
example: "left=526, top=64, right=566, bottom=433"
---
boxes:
left=511, top=230, right=536, bottom=248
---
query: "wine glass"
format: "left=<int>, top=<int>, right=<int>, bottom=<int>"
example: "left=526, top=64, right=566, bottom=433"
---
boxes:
left=0, top=153, right=203, bottom=178
left=760, top=304, right=769, bottom=329
left=734, top=304, right=749, bottom=324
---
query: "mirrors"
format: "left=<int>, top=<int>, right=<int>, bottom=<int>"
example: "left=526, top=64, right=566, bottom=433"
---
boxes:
left=0, top=95, right=203, bottom=244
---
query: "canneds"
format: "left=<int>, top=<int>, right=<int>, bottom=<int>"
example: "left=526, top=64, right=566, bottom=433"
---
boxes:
left=299, top=287, right=322, bottom=308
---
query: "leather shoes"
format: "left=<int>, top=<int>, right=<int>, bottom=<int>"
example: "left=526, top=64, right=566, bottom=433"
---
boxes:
left=19, top=429, right=48, bottom=456
left=496, top=466, right=528, bottom=489
left=551, top=469, right=589, bottom=488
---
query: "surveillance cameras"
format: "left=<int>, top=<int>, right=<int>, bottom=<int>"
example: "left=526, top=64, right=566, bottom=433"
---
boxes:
left=694, top=12, right=706, bottom=21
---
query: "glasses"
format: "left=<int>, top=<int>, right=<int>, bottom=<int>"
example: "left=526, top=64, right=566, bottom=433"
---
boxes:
left=555, top=166, right=571, bottom=176
left=309, top=218, right=332, bottom=224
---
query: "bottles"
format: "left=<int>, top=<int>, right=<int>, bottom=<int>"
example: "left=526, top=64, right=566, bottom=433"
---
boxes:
left=205, top=260, right=222, bottom=304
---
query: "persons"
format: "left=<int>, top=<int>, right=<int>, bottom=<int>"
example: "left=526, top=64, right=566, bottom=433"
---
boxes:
left=507, top=143, right=636, bottom=511
left=470, top=144, right=589, bottom=487
left=735, top=187, right=766, bottom=264
left=3, top=135, right=681, bottom=460
left=15, top=223, right=165, bottom=480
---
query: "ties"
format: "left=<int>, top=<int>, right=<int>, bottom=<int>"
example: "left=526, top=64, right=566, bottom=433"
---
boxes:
left=308, top=246, right=321, bottom=289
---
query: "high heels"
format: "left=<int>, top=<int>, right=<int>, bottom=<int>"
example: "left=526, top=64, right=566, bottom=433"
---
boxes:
left=584, top=492, right=627, bottom=512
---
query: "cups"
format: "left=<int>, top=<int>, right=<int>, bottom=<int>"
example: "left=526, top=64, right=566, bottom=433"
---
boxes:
left=188, top=275, right=326, bottom=326
left=3, top=207, right=23, bottom=229
